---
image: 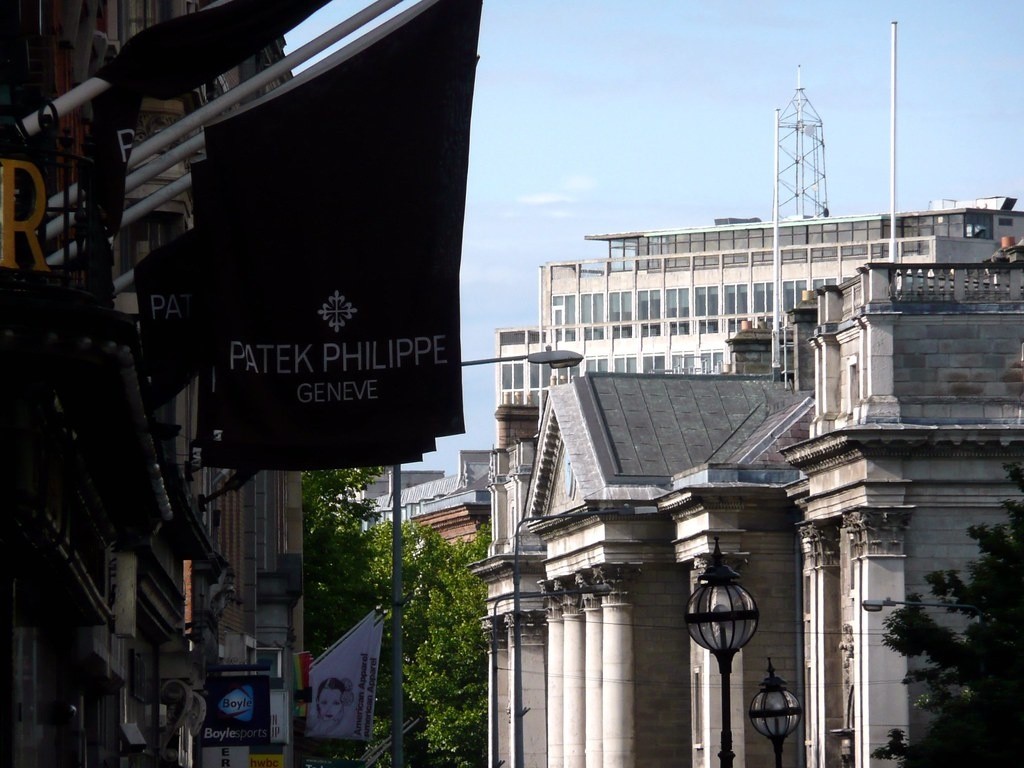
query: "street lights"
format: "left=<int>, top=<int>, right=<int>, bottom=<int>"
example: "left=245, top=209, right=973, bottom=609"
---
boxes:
left=514, top=506, right=660, bottom=768
left=391, top=345, right=585, bottom=768
left=749, top=657, right=802, bottom=768
left=685, top=537, right=758, bottom=768
left=492, top=584, right=612, bottom=768
left=861, top=598, right=984, bottom=698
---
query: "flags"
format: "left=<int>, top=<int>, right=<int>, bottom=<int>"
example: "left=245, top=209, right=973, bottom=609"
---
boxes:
left=71, top=1, right=480, bottom=468
left=306, top=614, right=383, bottom=743
